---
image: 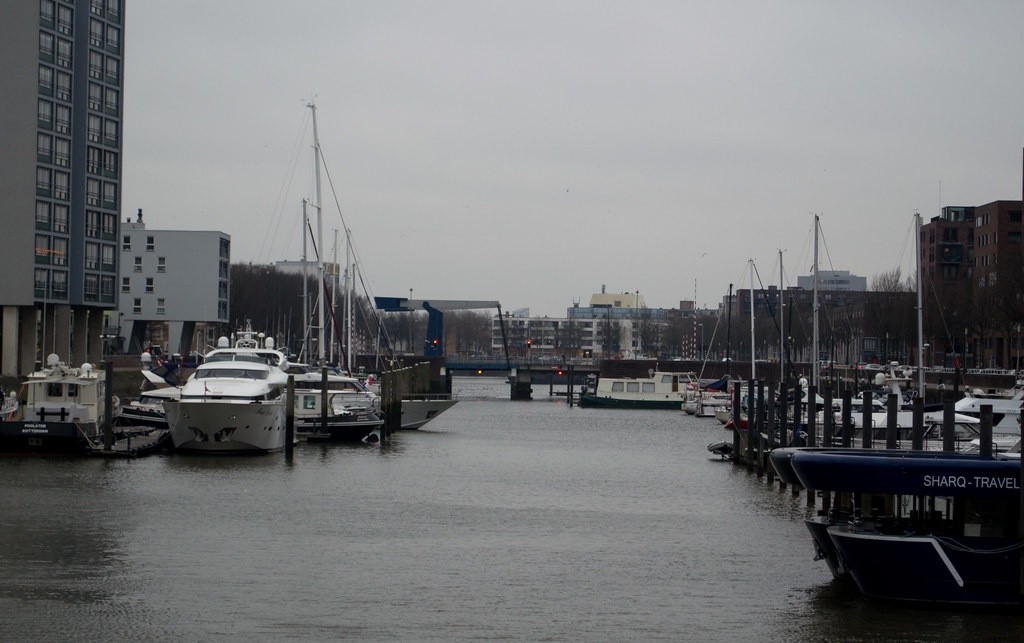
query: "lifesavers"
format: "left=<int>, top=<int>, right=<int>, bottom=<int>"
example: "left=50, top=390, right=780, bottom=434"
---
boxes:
left=694, top=385, right=698, bottom=391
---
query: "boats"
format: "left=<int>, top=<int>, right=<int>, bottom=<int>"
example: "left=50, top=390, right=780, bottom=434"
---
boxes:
left=952, top=348, right=1024, bottom=435
left=816, top=411, right=1024, bottom=460
left=161, top=316, right=299, bottom=455
left=118, top=336, right=186, bottom=430
left=0, top=267, right=123, bottom=457
left=768, top=445, right=1024, bottom=618
left=578, top=372, right=700, bottom=410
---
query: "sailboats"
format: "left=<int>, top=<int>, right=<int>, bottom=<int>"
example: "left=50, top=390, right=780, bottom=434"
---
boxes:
left=243, top=92, right=460, bottom=441
left=681, top=208, right=966, bottom=439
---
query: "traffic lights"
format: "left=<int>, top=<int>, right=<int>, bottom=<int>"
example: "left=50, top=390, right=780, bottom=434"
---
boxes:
left=528, top=340, right=531, bottom=348
